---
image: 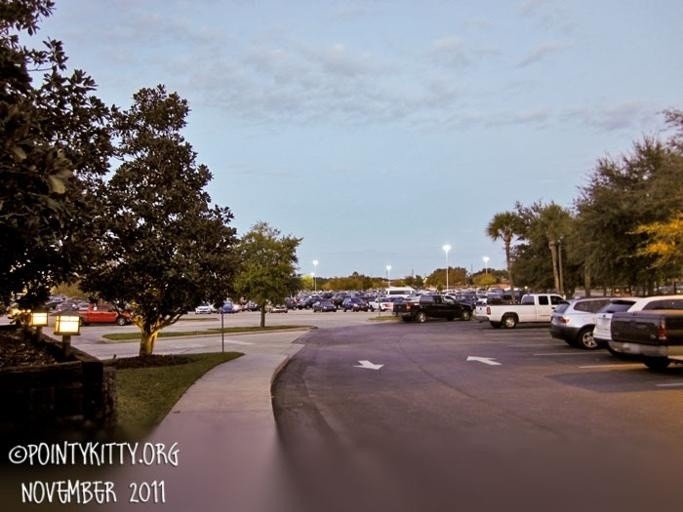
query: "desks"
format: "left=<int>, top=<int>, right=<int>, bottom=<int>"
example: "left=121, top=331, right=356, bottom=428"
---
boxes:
left=441, top=242, right=452, bottom=290
left=481, top=255, right=489, bottom=276
left=384, top=264, right=392, bottom=287
left=312, top=259, right=318, bottom=292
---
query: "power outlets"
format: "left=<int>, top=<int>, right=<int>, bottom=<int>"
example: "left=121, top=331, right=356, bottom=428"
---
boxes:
left=72, top=303, right=133, bottom=326
left=473, top=292, right=567, bottom=329
left=608, top=309, right=683, bottom=369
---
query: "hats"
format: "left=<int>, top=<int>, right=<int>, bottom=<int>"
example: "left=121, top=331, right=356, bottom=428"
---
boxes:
left=392, top=292, right=473, bottom=322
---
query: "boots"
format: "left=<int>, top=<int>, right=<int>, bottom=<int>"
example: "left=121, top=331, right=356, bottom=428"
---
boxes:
left=592, top=294, right=683, bottom=355
left=549, top=296, right=639, bottom=349
